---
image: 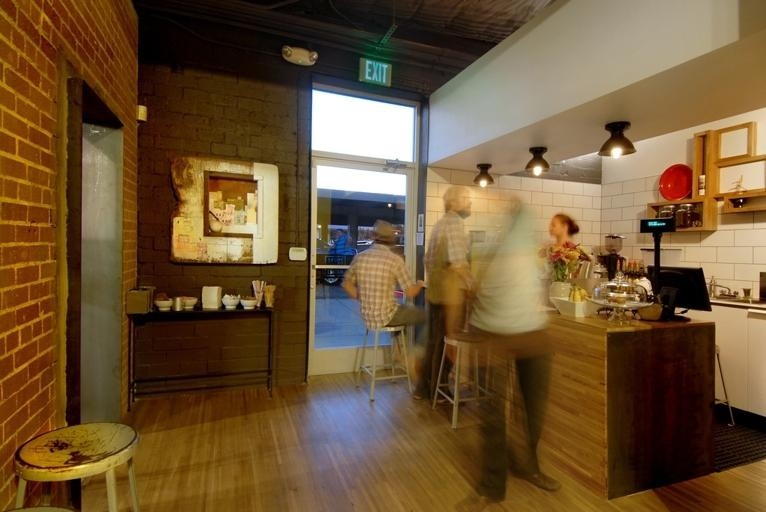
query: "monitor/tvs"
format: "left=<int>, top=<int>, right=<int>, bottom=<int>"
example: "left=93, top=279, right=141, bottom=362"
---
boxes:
left=647, top=264, right=712, bottom=323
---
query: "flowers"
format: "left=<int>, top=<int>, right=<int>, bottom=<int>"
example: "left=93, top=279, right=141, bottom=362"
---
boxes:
left=535, top=241, right=584, bottom=283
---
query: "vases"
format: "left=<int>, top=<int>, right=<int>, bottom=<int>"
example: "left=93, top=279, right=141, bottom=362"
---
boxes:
left=548, top=281, right=573, bottom=299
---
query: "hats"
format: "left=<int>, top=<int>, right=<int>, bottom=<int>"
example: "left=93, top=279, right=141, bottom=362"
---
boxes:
left=369, top=221, right=395, bottom=241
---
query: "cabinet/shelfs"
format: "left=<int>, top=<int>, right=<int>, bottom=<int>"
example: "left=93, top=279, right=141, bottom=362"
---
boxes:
left=647, top=121, right=766, bottom=230
left=460, top=291, right=717, bottom=500
left=676, top=295, right=766, bottom=417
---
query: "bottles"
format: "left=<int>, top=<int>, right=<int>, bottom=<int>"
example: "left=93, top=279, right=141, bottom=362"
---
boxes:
left=635, top=264, right=656, bottom=303
left=709, top=276, right=716, bottom=298
left=676, top=204, right=692, bottom=228
left=658, top=204, right=676, bottom=218
left=623, top=260, right=639, bottom=305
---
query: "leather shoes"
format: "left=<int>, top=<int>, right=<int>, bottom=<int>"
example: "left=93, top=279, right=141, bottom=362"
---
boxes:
left=455, top=493, right=503, bottom=512
left=522, top=472, right=561, bottom=491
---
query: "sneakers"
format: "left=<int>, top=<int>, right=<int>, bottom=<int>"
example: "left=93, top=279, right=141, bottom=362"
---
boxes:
left=434, top=396, right=466, bottom=407
left=410, top=391, right=423, bottom=402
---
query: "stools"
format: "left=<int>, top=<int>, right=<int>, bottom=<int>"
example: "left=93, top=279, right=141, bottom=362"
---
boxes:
left=356, top=323, right=413, bottom=402
left=14, top=420, right=142, bottom=512
left=430, top=331, right=490, bottom=429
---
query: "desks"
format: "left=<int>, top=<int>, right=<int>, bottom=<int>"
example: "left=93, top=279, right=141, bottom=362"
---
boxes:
left=126, top=301, right=277, bottom=414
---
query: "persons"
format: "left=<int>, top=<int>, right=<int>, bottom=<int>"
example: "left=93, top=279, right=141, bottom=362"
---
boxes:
left=414, top=189, right=481, bottom=405
left=342, top=220, right=434, bottom=327
left=326, top=229, right=357, bottom=262
left=539, top=212, right=580, bottom=256
left=457, top=172, right=559, bottom=511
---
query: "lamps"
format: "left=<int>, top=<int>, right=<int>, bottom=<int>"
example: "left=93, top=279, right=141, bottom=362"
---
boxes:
left=525, top=146, right=551, bottom=177
left=597, top=119, right=638, bottom=160
left=281, top=44, right=318, bottom=65
left=472, top=162, right=495, bottom=188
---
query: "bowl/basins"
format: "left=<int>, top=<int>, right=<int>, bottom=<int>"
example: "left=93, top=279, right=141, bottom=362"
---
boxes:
left=241, top=300, right=257, bottom=310
left=549, top=296, right=597, bottom=318
left=153, top=300, right=174, bottom=311
left=183, top=297, right=198, bottom=309
left=222, top=298, right=240, bottom=309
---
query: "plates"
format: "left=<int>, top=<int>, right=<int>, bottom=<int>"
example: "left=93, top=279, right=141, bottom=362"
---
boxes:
left=658, top=162, right=695, bottom=202
